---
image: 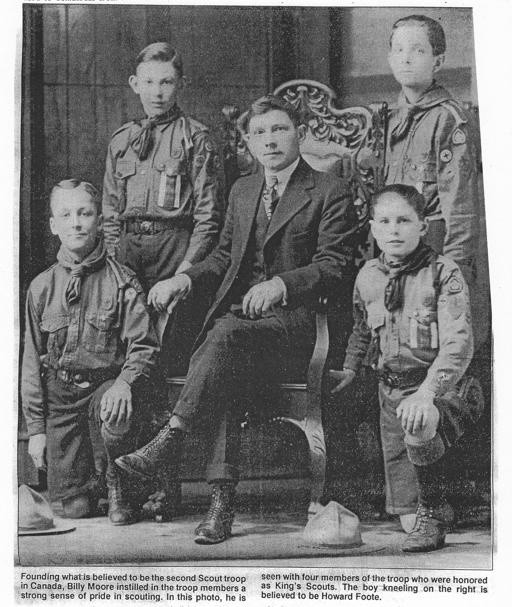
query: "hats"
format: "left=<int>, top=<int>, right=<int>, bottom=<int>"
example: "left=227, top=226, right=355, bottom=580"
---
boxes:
left=289, top=501, right=385, bottom=555
left=19, top=485, right=76, bottom=536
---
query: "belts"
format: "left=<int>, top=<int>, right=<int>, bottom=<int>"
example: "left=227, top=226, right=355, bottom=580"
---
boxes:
left=48, top=366, right=117, bottom=383
left=126, top=219, right=188, bottom=235
left=376, top=367, right=430, bottom=390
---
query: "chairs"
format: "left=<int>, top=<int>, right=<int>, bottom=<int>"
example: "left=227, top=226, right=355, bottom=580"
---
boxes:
left=142, top=79, right=388, bottom=524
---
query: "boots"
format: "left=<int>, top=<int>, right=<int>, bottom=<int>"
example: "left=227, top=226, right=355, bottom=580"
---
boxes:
left=114, top=423, right=188, bottom=478
left=194, top=484, right=237, bottom=544
left=403, top=492, right=454, bottom=552
left=106, top=473, right=139, bottom=525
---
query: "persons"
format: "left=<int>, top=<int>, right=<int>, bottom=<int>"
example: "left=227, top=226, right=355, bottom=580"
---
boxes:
left=383, top=15, right=475, bottom=275
left=101, top=43, right=221, bottom=520
left=21, top=179, right=162, bottom=526
left=327, top=184, right=473, bottom=551
left=115, top=96, right=359, bottom=546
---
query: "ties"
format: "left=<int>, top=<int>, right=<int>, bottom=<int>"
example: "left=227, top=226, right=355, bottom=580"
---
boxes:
left=263, top=176, right=280, bottom=222
